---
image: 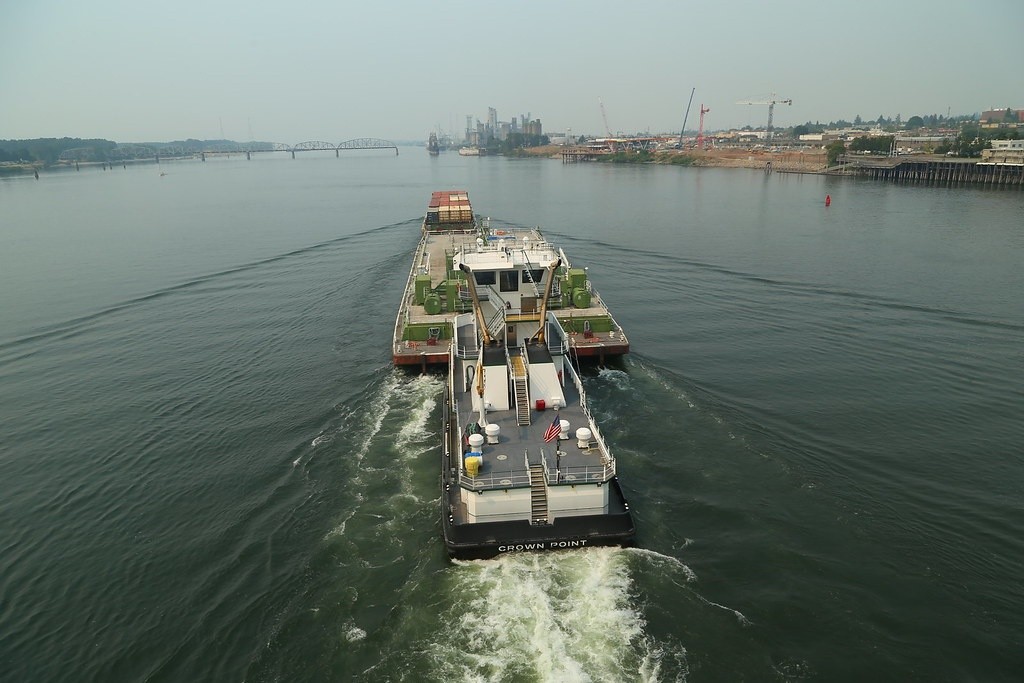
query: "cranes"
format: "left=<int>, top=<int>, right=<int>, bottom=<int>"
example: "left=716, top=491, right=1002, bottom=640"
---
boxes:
left=735, top=94, right=792, bottom=146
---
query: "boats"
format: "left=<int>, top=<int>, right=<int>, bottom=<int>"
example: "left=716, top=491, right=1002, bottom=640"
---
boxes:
left=388, top=182, right=645, bottom=561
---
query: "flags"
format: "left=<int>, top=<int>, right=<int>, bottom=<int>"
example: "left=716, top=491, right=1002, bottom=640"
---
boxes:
left=542, top=414, right=562, bottom=445
